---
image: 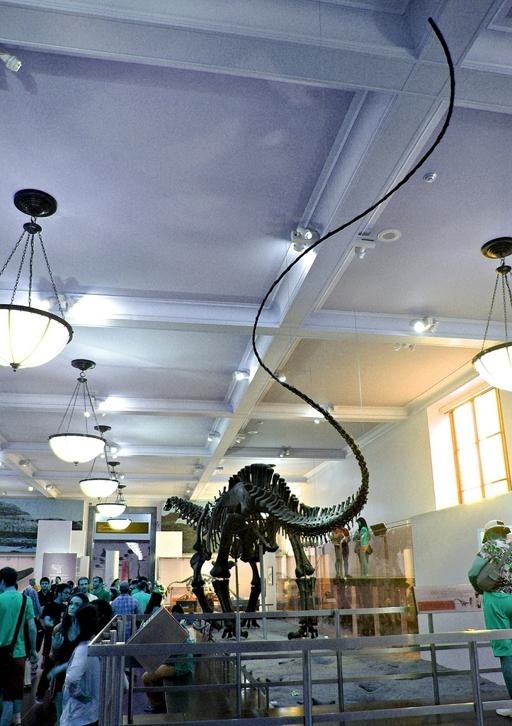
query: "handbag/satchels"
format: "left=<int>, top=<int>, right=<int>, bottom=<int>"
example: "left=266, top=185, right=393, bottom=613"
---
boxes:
left=477, top=562, right=501, bottom=592
left=0, top=646, right=12, bottom=678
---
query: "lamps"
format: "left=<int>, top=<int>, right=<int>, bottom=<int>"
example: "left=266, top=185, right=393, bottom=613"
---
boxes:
left=1, top=44, right=24, bottom=74
left=78, top=424, right=120, bottom=501
left=178, top=366, right=338, bottom=497
left=0, top=189, right=74, bottom=373
left=471, top=233, right=511, bottom=396
left=97, top=462, right=127, bottom=521
left=284, top=223, right=320, bottom=254
left=48, top=359, right=106, bottom=466
left=108, top=485, right=132, bottom=531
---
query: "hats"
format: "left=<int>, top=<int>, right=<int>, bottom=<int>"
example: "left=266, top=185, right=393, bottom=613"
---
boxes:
left=482, top=520, right=510, bottom=543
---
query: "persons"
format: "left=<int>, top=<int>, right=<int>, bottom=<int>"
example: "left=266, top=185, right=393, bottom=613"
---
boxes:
left=0, top=566, right=195, bottom=726
left=333, top=525, right=352, bottom=579
left=468, top=519, right=512, bottom=719
left=353, top=517, right=371, bottom=577
